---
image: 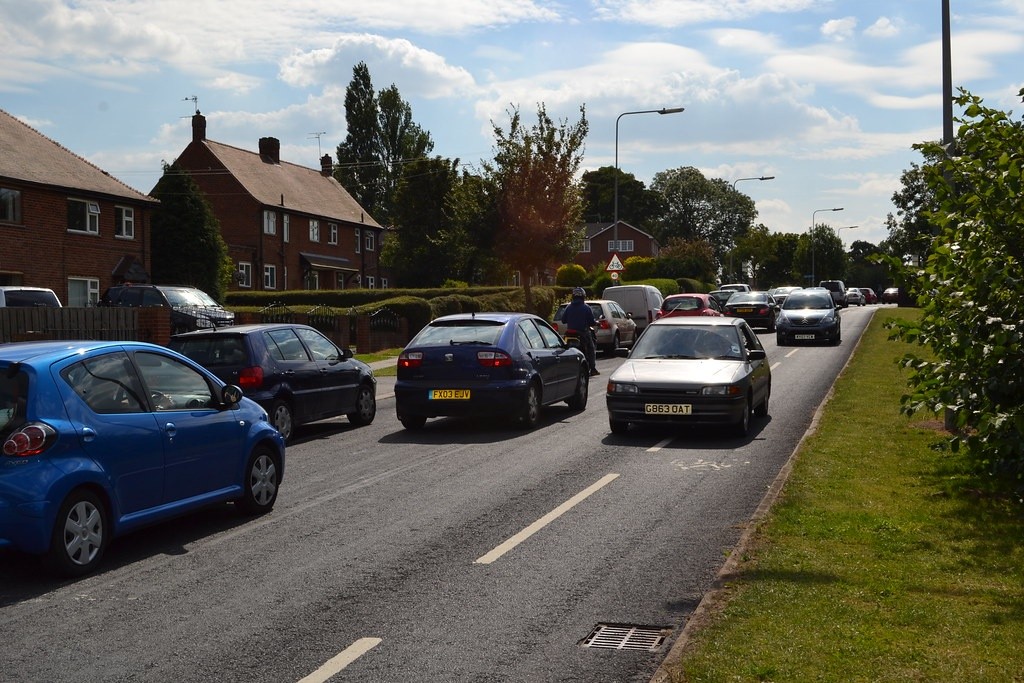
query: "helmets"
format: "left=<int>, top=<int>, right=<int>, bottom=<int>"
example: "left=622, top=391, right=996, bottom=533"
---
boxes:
left=572, top=287, right=586, bottom=297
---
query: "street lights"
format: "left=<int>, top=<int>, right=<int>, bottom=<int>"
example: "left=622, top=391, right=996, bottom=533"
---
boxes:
left=812, top=208, right=843, bottom=287
left=615, top=108, right=685, bottom=284
left=730, top=176, right=775, bottom=285
left=838, top=226, right=859, bottom=238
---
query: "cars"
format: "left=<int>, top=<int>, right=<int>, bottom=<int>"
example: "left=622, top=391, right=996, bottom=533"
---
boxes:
left=723, top=291, right=783, bottom=332
left=881, top=287, right=898, bottom=304
left=860, top=288, right=878, bottom=304
left=394, top=311, right=589, bottom=431
left=709, top=284, right=804, bottom=307
left=845, top=288, right=866, bottom=306
left=553, top=300, right=638, bottom=353
left=165, top=323, right=377, bottom=441
left=0, top=340, right=286, bottom=580
left=607, top=316, right=771, bottom=437
left=655, top=293, right=724, bottom=321
left=776, top=288, right=842, bottom=345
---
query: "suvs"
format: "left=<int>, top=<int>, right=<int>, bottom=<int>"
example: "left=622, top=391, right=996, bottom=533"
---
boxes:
left=819, top=280, right=849, bottom=308
left=97, top=283, right=235, bottom=335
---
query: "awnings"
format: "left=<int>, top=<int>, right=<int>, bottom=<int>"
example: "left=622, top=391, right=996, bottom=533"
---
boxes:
left=300, top=252, right=359, bottom=272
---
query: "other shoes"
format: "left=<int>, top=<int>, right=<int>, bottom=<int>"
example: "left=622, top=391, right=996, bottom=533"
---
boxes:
left=591, top=367, right=600, bottom=375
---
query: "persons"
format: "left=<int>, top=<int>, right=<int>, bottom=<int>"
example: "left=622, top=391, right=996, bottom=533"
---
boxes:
left=562, top=287, right=600, bottom=375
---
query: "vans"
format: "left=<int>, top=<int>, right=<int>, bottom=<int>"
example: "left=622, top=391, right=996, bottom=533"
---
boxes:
left=0, top=286, right=62, bottom=308
left=603, top=285, right=665, bottom=332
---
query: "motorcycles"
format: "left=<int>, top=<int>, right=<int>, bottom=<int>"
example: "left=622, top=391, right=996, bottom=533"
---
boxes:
left=563, top=314, right=603, bottom=351
